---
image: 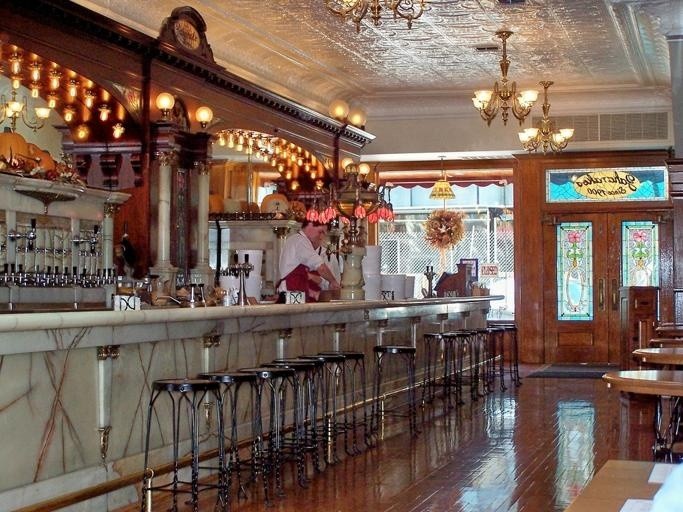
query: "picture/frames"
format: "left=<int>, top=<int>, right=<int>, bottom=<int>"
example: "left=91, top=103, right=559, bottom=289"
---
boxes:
left=460, top=259, right=478, bottom=281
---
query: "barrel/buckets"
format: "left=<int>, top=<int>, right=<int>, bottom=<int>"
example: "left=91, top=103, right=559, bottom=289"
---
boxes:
left=405, top=277, right=414, bottom=297
left=219, top=276, right=240, bottom=291
left=381, top=274, right=407, bottom=301
left=243, top=276, right=262, bottom=301
left=364, top=274, right=383, bottom=300
left=236, top=249, right=262, bottom=276
left=362, top=246, right=381, bottom=274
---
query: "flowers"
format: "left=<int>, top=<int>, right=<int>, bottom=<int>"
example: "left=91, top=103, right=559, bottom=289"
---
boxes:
left=423, top=209, right=465, bottom=248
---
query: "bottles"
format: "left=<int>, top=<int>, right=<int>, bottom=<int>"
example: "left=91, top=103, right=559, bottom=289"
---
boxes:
left=223, top=287, right=239, bottom=306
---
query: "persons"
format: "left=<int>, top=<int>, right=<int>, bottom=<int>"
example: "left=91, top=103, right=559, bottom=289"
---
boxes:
left=273, top=217, right=340, bottom=304
left=315, top=245, right=341, bottom=289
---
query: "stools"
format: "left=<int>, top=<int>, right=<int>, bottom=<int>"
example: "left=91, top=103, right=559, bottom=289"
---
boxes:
left=422, top=320, right=523, bottom=413
left=372, top=345, right=422, bottom=441
left=142, top=351, right=370, bottom=512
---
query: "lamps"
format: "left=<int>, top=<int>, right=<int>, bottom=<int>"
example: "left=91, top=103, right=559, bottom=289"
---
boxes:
left=196, top=107, right=213, bottom=133
left=329, top=100, right=349, bottom=123
left=305, top=173, right=396, bottom=246
left=0, top=52, right=125, bottom=141
left=429, top=156, right=456, bottom=201
left=347, top=108, right=366, bottom=130
left=325, top=0, right=426, bottom=34
left=472, top=30, right=539, bottom=126
left=518, top=80, right=575, bottom=155
left=156, top=92, right=175, bottom=122
left=228, top=130, right=273, bottom=152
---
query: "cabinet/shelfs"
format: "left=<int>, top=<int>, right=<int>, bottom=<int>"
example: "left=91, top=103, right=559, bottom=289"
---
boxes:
left=618, top=285, right=662, bottom=408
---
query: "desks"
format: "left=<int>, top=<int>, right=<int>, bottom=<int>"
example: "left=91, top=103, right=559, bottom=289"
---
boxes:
left=564, top=460, right=683, bottom=512
left=602, top=370, right=683, bottom=461
left=631, top=318, right=683, bottom=370
